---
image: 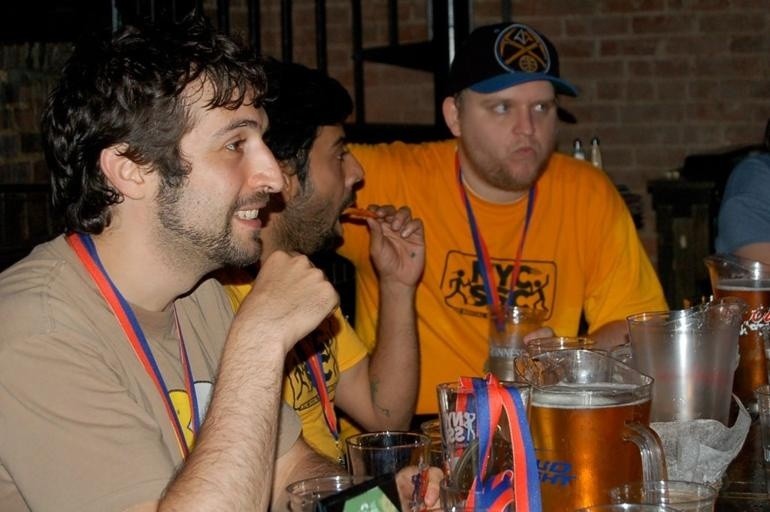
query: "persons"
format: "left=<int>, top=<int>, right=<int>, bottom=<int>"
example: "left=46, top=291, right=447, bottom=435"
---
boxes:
left=0, top=16, right=445, bottom=512
left=216, top=58, right=427, bottom=473
left=332, top=20, right=670, bottom=440
left=713, top=116, right=770, bottom=280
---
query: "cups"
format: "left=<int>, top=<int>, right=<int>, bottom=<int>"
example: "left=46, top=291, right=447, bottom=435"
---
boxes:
left=514, top=346, right=667, bottom=512
left=482, top=300, right=542, bottom=389
left=609, top=480, right=719, bottom=512
left=283, top=478, right=375, bottom=512
left=343, top=430, right=434, bottom=512
left=528, top=332, right=600, bottom=355
left=435, top=378, right=530, bottom=512
left=708, top=256, right=768, bottom=406
left=624, top=304, right=741, bottom=499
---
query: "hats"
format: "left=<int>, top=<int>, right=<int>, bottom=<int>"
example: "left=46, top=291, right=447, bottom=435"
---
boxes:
left=448, top=22, right=579, bottom=97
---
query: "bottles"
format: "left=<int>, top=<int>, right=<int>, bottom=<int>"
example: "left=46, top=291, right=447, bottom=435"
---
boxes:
left=573, top=137, right=603, bottom=171
